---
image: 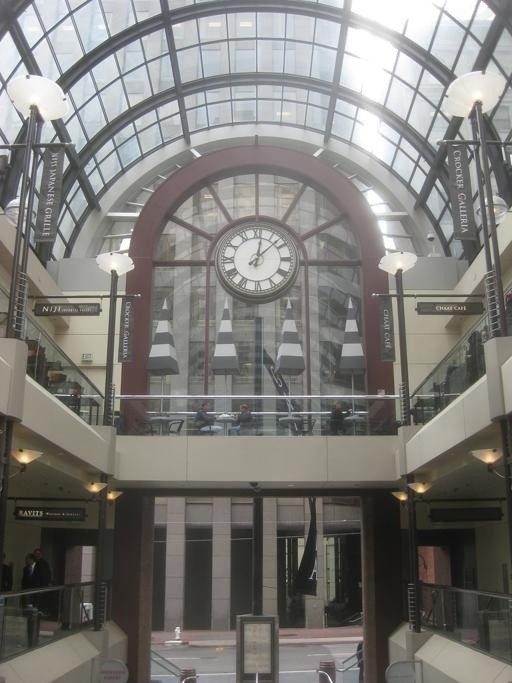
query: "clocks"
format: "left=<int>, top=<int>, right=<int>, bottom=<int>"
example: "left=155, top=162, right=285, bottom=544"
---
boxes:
left=214, top=223, right=299, bottom=303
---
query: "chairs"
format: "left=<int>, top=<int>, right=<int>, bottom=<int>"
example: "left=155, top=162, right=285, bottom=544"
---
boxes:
left=2, top=314, right=99, bottom=425
left=395, top=329, right=485, bottom=429
left=110, top=403, right=369, bottom=436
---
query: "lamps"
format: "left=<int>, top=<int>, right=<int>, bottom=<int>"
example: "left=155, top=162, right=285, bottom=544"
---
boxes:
left=83, top=482, right=108, bottom=501
left=105, top=491, right=123, bottom=506
left=471, top=447, right=512, bottom=479
left=392, top=491, right=408, bottom=511
left=408, top=482, right=432, bottom=501
left=9, top=449, right=43, bottom=479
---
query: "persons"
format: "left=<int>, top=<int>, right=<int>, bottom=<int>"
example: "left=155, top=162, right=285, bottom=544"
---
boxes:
left=20, top=553, right=42, bottom=617
left=330, top=400, right=352, bottom=436
left=0, top=550, right=14, bottom=605
left=195, top=402, right=224, bottom=436
left=467, top=330, right=486, bottom=381
left=480, top=329, right=487, bottom=341
left=228, top=403, right=253, bottom=436
left=33, top=548, right=52, bottom=595
